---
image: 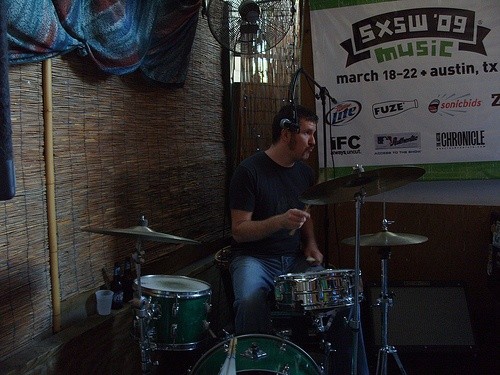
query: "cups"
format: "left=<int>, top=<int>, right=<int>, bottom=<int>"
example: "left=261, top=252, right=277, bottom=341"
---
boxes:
left=95, top=290, right=114, bottom=315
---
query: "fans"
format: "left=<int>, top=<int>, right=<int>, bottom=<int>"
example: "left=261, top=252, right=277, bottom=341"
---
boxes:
left=206, top=0, right=294, bottom=55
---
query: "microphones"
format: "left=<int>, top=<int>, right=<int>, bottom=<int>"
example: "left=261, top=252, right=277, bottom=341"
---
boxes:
left=279, top=118, right=300, bottom=130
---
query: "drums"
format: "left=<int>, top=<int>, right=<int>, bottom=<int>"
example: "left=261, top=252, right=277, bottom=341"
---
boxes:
left=273, top=268, right=363, bottom=312
left=129, top=274, right=212, bottom=351
left=185, top=334, right=324, bottom=375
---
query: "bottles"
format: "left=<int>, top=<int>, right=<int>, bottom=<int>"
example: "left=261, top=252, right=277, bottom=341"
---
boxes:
left=123, top=257, right=134, bottom=303
left=110, top=263, right=124, bottom=310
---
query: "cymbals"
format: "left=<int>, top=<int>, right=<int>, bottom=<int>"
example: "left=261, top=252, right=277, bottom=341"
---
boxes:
left=342, top=231, right=429, bottom=246
left=81, top=224, right=201, bottom=244
left=298, top=166, right=426, bottom=205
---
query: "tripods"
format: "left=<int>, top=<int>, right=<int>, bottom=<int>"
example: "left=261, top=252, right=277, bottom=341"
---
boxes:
left=344, top=179, right=408, bottom=375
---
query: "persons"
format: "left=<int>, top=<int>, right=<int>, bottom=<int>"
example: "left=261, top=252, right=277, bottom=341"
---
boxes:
left=229, top=105, right=369, bottom=375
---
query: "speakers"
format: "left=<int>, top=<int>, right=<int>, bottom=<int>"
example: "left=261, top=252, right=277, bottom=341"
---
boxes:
left=369, top=279, right=476, bottom=375
